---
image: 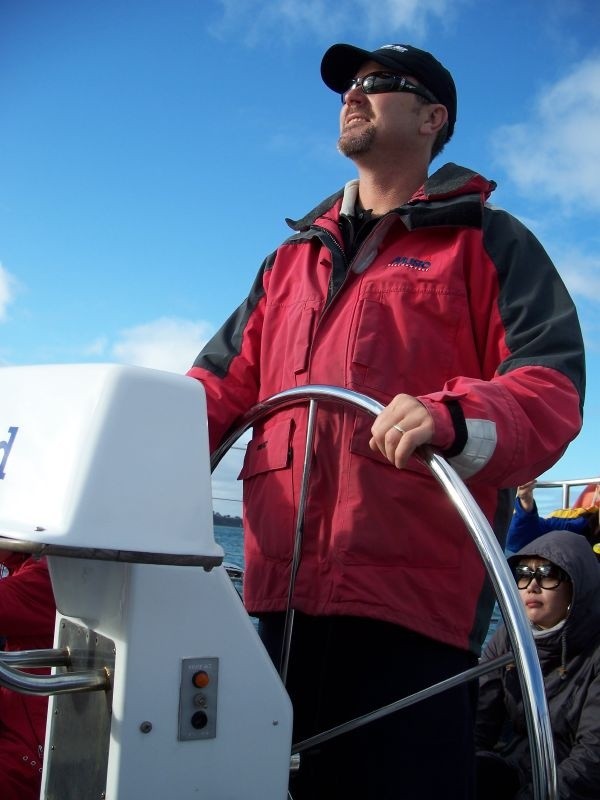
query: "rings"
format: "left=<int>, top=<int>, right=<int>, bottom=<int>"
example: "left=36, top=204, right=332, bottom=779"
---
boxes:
left=394, top=425, right=406, bottom=435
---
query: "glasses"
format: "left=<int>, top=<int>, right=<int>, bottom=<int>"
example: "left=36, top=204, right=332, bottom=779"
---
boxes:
left=512, top=565, right=568, bottom=589
left=342, top=70, right=449, bottom=128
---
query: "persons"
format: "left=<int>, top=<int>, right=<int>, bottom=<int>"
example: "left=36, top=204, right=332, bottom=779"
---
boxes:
left=0, top=549, right=56, bottom=800
left=185, top=44, right=588, bottom=800
left=470, top=480, right=600, bottom=800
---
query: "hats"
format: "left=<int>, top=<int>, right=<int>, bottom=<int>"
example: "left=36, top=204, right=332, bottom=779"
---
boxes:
left=319, top=43, right=457, bottom=133
